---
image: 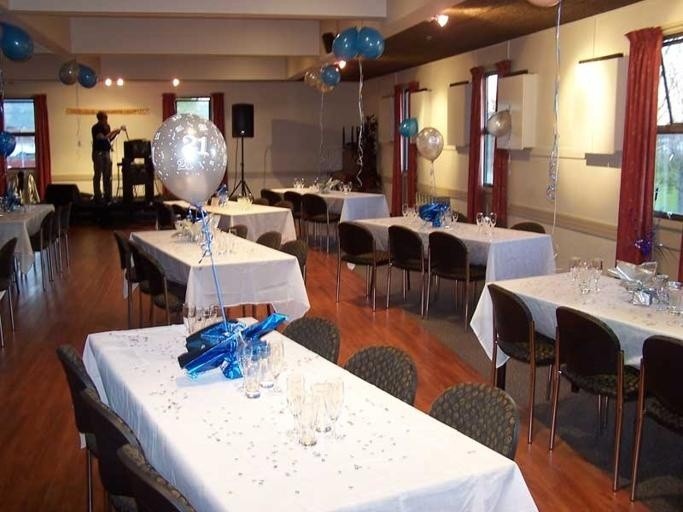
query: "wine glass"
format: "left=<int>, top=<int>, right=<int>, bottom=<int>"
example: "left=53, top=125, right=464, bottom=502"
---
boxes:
left=590, top=257, right=603, bottom=295
left=324, top=376, right=344, bottom=444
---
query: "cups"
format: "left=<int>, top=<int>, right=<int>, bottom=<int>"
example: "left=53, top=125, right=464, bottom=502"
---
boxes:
left=567, top=255, right=592, bottom=295
left=292, top=176, right=353, bottom=196
left=401, top=203, right=458, bottom=230
left=475, top=211, right=497, bottom=240
left=173, top=214, right=237, bottom=256
left=177, top=303, right=217, bottom=335
left=241, top=336, right=283, bottom=402
left=653, top=273, right=683, bottom=316
left=278, top=372, right=345, bottom=448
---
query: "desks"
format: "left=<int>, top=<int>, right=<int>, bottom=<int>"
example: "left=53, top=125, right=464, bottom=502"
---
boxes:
left=485, top=269, right=683, bottom=392
left=271, top=187, right=385, bottom=248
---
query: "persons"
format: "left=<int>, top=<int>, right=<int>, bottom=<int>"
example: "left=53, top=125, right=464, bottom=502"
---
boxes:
left=90, top=110, right=127, bottom=206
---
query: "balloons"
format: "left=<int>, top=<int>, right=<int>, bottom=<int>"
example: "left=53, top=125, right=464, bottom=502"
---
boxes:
left=146, top=109, right=230, bottom=212
left=413, top=127, right=446, bottom=165
left=0, top=131, right=17, bottom=160
left=484, top=109, right=512, bottom=137
left=399, top=119, right=420, bottom=138
left=56, top=59, right=81, bottom=84
left=1, top=23, right=35, bottom=64
left=76, top=68, right=98, bottom=90
left=300, top=22, right=385, bottom=93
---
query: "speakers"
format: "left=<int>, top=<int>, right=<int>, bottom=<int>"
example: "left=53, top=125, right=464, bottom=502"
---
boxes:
left=231, top=103, right=255, bottom=138
left=45, top=184, right=78, bottom=201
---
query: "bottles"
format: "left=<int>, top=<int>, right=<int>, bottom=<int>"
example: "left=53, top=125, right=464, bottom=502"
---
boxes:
left=218, top=184, right=229, bottom=208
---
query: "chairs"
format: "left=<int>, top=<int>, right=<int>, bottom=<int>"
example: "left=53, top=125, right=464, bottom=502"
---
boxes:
left=260, top=187, right=342, bottom=255
left=487, top=284, right=683, bottom=502
left=336, top=209, right=551, bottom=332
left=112, top=193, right=309, bottom=330
left=56, top=316, right=519, bottom=511
left=0, top=182, right=78, bottom=350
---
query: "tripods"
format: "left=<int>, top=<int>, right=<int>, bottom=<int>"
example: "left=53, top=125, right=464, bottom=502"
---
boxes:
left=230, top=141, right=253, bottom=199
